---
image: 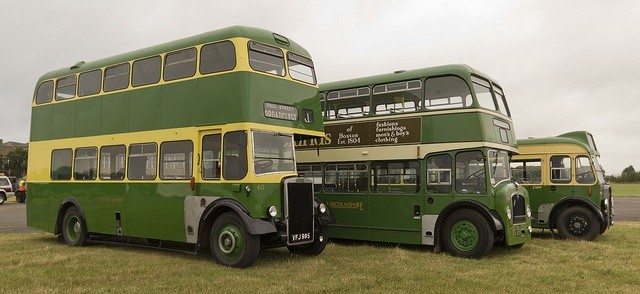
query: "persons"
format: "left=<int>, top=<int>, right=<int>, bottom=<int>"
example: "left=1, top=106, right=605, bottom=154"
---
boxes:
left=18, top=177, right=26, bottom=203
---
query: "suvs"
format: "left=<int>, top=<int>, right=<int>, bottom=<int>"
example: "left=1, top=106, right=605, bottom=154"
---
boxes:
left=14, top=178, right=26, bottom=203
left=0, top=176, right=15, bottom=204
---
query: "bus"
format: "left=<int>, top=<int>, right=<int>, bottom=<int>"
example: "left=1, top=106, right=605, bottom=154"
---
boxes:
left=295, top=63, right=532, bottom=258
left=26, top=25, right=335, bottom=269
left=511, top=131, right=614, bottom=242
